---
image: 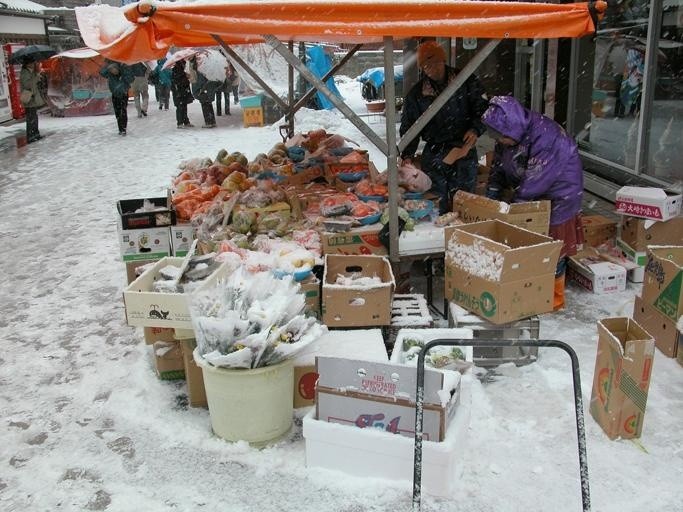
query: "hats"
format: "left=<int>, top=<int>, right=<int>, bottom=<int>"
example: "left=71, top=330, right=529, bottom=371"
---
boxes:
left=417, top=41, right=447, bottom=67
left=22, top=55, right=36, bottom=68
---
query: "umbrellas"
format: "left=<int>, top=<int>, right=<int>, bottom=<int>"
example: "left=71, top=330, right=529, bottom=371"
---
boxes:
left=6, top=43, right=59, bottom=66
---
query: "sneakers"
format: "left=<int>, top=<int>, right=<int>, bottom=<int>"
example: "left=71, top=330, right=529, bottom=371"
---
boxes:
left=119, top=128, right=127, bottom=135
left=138, top=110, right=147, bottom=117
left=177, top=123, right=216, bottom=128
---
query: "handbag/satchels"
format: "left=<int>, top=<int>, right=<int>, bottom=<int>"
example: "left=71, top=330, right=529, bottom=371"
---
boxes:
left=20, top=89, right=33, bottom=104
left=184, top=61, right=197, bottom=84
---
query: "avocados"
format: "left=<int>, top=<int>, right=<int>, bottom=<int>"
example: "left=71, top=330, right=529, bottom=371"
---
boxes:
left=210, top=211, right=289, bottom=251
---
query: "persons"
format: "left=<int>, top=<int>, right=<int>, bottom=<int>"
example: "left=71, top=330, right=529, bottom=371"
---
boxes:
left=398, top=39, right=487, bottom=279
left=19, top=58, right=45, bottom=143
left=99, top=49, right=239, bottom=136
left=478, top=93, right=586, bottom=311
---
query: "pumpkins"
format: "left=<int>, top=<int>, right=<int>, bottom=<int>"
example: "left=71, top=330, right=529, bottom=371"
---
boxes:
left=200, top=142, right=288, bottom=173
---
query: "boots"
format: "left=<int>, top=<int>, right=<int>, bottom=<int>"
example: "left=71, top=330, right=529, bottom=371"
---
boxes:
left=553, top=273, right=565, bottom=311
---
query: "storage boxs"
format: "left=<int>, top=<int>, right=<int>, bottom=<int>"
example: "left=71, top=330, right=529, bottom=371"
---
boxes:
left=453, top=190, right=552, bottom=236
left=115, top=190, right=474, bottom=495
left=445, top=218, right=564, bottom=325
left=72, top=91, right=90, bottom=100
left=567, top=186, right=683, bottom=441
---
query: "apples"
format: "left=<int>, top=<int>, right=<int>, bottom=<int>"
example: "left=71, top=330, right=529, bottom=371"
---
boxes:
left=280, top=250, right=315, bottom=267
left=324, top=183, right=387, bottom=218
